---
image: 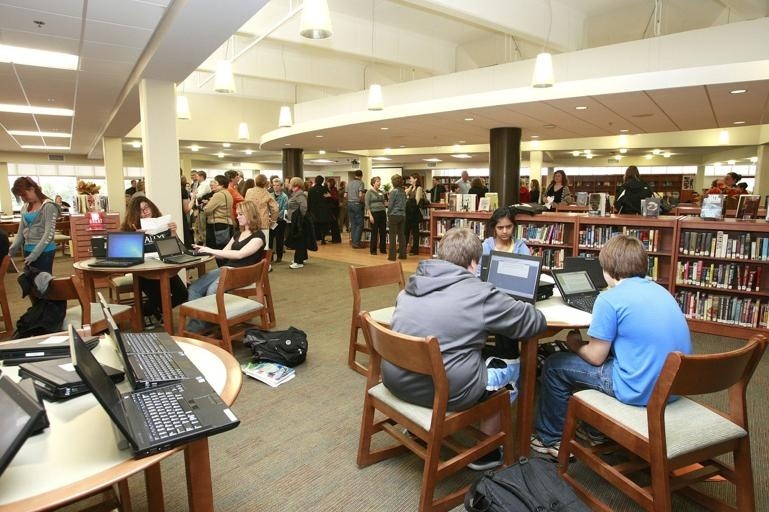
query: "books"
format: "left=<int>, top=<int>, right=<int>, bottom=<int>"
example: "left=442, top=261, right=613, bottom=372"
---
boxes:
left=670, top=193, right=769, bottom=331
left=518, top=192, right=660, bottom=282
left=241, top=360, right=296, bottom=388
left=485, top=191, right=499, bottom=210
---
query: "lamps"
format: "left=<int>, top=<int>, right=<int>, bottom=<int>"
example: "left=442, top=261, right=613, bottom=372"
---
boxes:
left=365, top=73, right=386, bottom=115
left=238, top=120, right=250, bottom=142
left=212, top=54, right=238, bottom=95
left=277, top=92, right=292, bottom=129
left=175, top=86, right=193, bottom=123
left=296, top=0, right=336, bottom=42
left=530, top=38, right=554, bottom=93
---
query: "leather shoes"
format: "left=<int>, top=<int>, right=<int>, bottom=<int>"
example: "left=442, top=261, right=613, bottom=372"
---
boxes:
left=351, top=244, right=418, bottom=262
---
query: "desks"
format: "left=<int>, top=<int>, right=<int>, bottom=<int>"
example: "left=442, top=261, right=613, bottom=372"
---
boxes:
left=0, top=210, right=70, bottom=256
left=1, top=331, right=243, bottom=512
left=72, top=249, right=216, bottom=335
left=513, top=284, right=593, bottom=460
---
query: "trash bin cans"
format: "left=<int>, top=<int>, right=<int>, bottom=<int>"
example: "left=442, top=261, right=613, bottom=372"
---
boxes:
left=91, top=236, right=107, bottom=257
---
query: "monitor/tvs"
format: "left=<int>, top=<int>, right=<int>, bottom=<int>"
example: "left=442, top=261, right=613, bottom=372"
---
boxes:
left=486, top=250, right=544, bottom=305
left=563, top=256, right=608, bottom=288
left=481, top=255, right=490, bottom=281
left=0, top=375, right=46, bottom=477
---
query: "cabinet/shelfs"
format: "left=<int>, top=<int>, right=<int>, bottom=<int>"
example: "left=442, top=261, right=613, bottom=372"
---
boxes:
left=429, top=211, right=767, bottom=338
left=352, top=200, right=445, bottom=257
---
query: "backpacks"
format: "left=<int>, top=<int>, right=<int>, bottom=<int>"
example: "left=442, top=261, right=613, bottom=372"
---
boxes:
left=464, top=455, right=590, bottom=512
left=243, top=326, right=308, bottom=365
left=11, top=301, right=64, bottom=340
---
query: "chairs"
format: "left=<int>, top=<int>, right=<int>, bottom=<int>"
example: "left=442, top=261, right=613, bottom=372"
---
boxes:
left=179, top=259, right=272, bottom=356
left=108, top=274, right=148, bottom=309
left=0, top=255, right=14, bottom=338
left=71, top=215, right=122, bottom=303
left=18, top=274, right=137, bottom=340
left=356, top=310, right=513, bottom=512
left=219, top=250, right=275, bottom=331
left=556, top=332, right=769, bottom=511
left=349, top=261, right=408, bottom=383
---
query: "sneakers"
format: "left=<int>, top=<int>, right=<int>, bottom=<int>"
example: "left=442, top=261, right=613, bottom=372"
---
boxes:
left=469, top=447, right=503, bottom=471
left=154, top=305, right=165, bottom=326
left=574, top=424, right=606, bottom=446
left=143, top=313, right=156, bottom=330
left=291, top=259, right=309, bottom=264
left=289, top=261, right=304, bottom=269
left=531, top=434, right=574, bottom=460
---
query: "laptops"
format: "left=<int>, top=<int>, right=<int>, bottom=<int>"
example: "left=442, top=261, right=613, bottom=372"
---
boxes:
left=88, top=231, right=145, bottom=267
left=0, top=335, right=99, bottom=366
left=97, top=291, right=184, bottom=353
left=102, top=308, right=205, bottom=390
left=550, top=267, right=600, bottom=314
left=175, top=235, right=205, bottom=256
left=153, top=236, right=201, bottom=264
left=67, top=323, right=241, bottom=460
left=19, top=356, right=125, bottom=403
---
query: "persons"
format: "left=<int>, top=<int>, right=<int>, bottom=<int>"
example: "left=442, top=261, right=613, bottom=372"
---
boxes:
left=710, top=173, right=751, bottom=197
left=119, top=196, right=187, bottom=331
left=9, top=177, right=62, bottom=277
left=0, top=228, right=8, bottom=264
left=615, top=164, right=653, bottom=214
left=474, top=205, right=530, bottom=278
left=54, top=196, right=70, bottom=212
left=126, top=177, right=146, bottom=197
left=181, top=161, right=490, bottom=271
left=519, top=170, right=571, bottom=213
left=188, top=202, right=267, bottom=332
left=381, top=230, right=546, bottom=472
left=530, top=234, right=693, bottom=457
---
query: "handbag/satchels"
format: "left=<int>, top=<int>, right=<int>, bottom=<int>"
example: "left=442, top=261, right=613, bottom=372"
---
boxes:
left=419, top=196, right=430, bottom=209
left=215, top=227, right=231, bottom=248
